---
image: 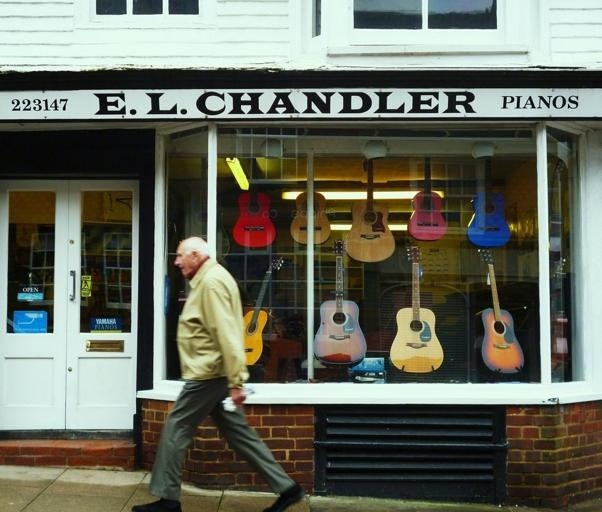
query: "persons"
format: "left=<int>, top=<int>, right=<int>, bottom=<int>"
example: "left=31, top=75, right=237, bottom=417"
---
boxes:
left=129, top=234, right=305, bottom=511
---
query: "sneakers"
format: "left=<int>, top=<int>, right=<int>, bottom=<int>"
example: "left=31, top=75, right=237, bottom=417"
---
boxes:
left=131, top=499, right=182, bottom=512
left=262, top=485, right=304, bottom=512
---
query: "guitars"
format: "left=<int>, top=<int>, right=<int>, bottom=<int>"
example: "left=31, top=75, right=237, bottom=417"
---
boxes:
left=408, top=158, right=447, bottom=241
left=181, top=156, right=230, bottom=263
left=389, top=245, right=445, bottom=376
left=233, top=158, right=277, bottom=248
left=343, top=160, right=396, bottom=263
left=465, top=158, right=511, bottom=249
left=473, top=248, right=524, bottom=371
left=243, top=256, right=286, bottom=371
left=290, top=191, right=331, bottom=245
left=314, top=238, right=367, bottom=368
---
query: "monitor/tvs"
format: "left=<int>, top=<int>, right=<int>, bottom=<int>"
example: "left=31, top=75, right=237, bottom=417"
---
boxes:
left=344, top=350, right=390, bottom=381
